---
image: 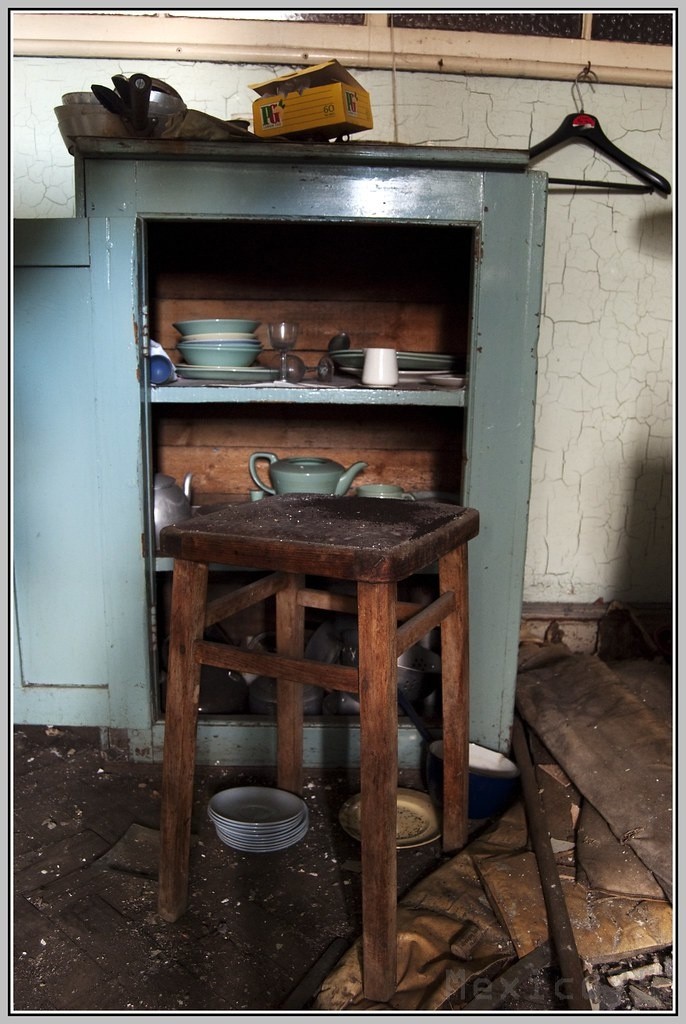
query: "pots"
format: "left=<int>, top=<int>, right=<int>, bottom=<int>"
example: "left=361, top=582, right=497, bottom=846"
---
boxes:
left=56, top=74, right=188, bottom=155
left=342, top=619, right=440, bottom=744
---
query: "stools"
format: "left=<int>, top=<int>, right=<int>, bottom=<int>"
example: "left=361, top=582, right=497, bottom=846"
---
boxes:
left=151, top=493, right=483, bottom=1003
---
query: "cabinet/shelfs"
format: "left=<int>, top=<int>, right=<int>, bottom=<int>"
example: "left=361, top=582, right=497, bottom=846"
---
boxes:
left=84, top=155, right=550, bottom=772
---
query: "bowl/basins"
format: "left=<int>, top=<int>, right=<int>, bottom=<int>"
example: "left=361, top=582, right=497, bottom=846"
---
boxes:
left=425, top=740, right=520, bottom=817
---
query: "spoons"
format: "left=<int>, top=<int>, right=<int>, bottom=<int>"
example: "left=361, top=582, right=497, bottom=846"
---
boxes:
left=329, top=333, right=351, bottom=351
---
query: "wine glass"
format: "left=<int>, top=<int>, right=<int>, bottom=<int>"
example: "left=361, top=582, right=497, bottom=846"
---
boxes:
left=268, top=321, right=297, bottom=384
left=272, top=354, right=334, bottom=384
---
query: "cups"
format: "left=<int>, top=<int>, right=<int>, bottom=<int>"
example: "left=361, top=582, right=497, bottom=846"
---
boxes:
left=363, top=347, right=398, bottom=392
left=358, top=484, right=415, bottom=501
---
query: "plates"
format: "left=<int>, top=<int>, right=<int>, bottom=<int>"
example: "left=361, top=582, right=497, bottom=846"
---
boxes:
left=340, top=788, right=444, bottom=849
left=330, top=346, right=467, bottom=391
left=207, top=786, right=310, bottom=850
left=173, top=320, right=280, bottom=379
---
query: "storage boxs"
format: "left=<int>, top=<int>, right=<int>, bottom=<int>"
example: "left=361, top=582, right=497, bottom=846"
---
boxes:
left=249, top=58, right=375, bottom=140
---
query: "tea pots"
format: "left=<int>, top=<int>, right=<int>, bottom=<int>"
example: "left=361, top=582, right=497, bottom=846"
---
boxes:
left=251, top=452, right=369, bottom=496
left=152, top=472, right=196, bottom=548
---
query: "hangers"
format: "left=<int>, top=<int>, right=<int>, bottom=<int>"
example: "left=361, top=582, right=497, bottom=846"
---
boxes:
left=527, top=70, right=672, bottom=196
